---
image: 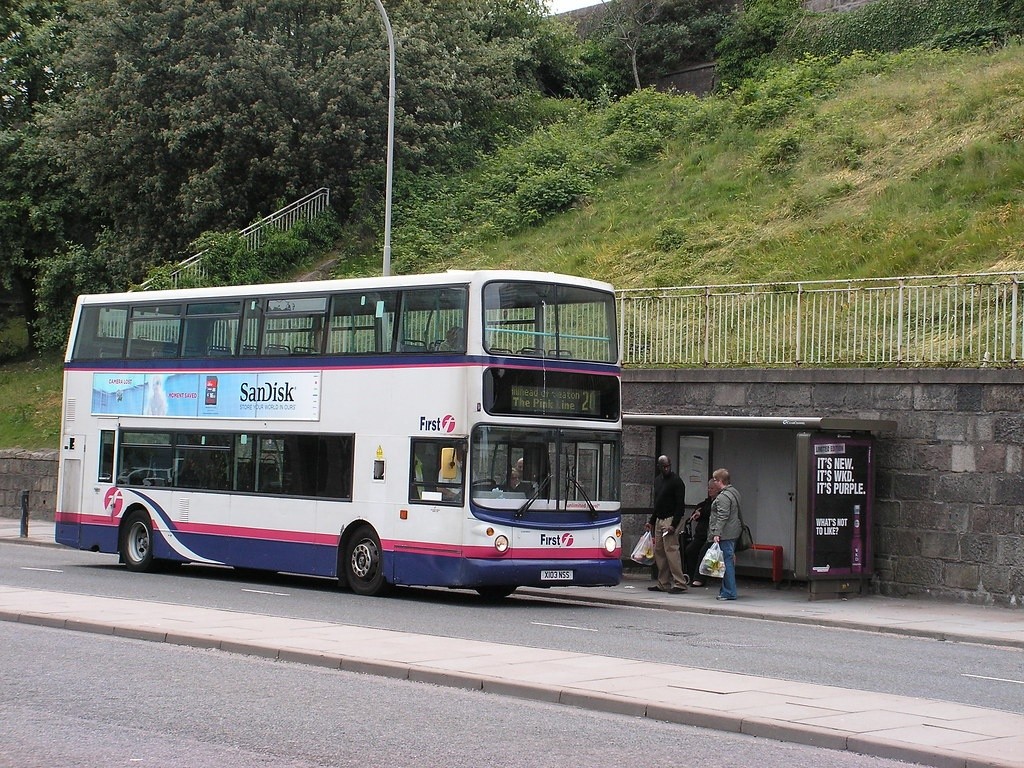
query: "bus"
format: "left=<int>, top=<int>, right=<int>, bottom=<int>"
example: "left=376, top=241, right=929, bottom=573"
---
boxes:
left=54, top=268, right=623, bottom=598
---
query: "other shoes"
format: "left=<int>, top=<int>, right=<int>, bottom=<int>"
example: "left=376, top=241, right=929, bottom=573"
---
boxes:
left=692, top=580, right=703, bottom=586
left=716, top=595, right=727, bottom=600
left=668, top=587, right=685, bottom=593
left=648, top=586, right=661, bottom=591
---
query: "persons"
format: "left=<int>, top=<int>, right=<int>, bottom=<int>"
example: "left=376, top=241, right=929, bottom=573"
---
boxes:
left=437, top=448, right=480, bottom=501
left=646, top=455, right=688, bottom=594
left=439, top=326, right=465, bottom=351
left=502, top=458, right=523, bottom=489
left=686, top=469, right=743, bottom=601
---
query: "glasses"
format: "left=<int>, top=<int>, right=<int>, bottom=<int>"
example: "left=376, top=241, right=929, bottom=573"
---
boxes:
left=712, top=479, right=717, bottom=482
left=512, top=477, right=520, bottom=480
left=660, top=464, right=672, bottom=469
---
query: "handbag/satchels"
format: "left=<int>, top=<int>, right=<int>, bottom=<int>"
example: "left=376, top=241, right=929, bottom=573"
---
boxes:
left=630, top=531, right=655, bottom=565
left=698, top=543, right=726, bottom=578
left=734, top=525, right=753, bottom=551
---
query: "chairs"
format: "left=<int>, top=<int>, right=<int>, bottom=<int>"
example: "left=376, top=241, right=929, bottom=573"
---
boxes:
left=259, top=458, right=276, bottom=486
left=421, top=454, right=439, bottom=492
left=399, top=339, right=428, bottom=352
left=266, top=343, right=291, bottom=354
left=489, top=348, right=513, bottom=355
left=130, top=345, right=155, bottom=357
left=207, top=345, right=232, bottom=355
left=293, top=347, right=318, bottom=354
left=259, top=454, right=280, bottom=492
left=151, top=454, right=172, bottom=486
left=521, top=347, right=546, bottom=357
left=242, top=345, right=258, bottom=355
left=548, top=350, right=573, bottom=358
left=238, top=458, right=252, bottom=490
left=151, top=346, right=177, bottom=357
left=516, top=350, right=536, bottom=355
left=100, top=342, right=123, bottom=358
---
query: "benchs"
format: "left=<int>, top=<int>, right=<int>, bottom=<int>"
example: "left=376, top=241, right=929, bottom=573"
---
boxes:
left=751, top=544, right=783, bottom=581
